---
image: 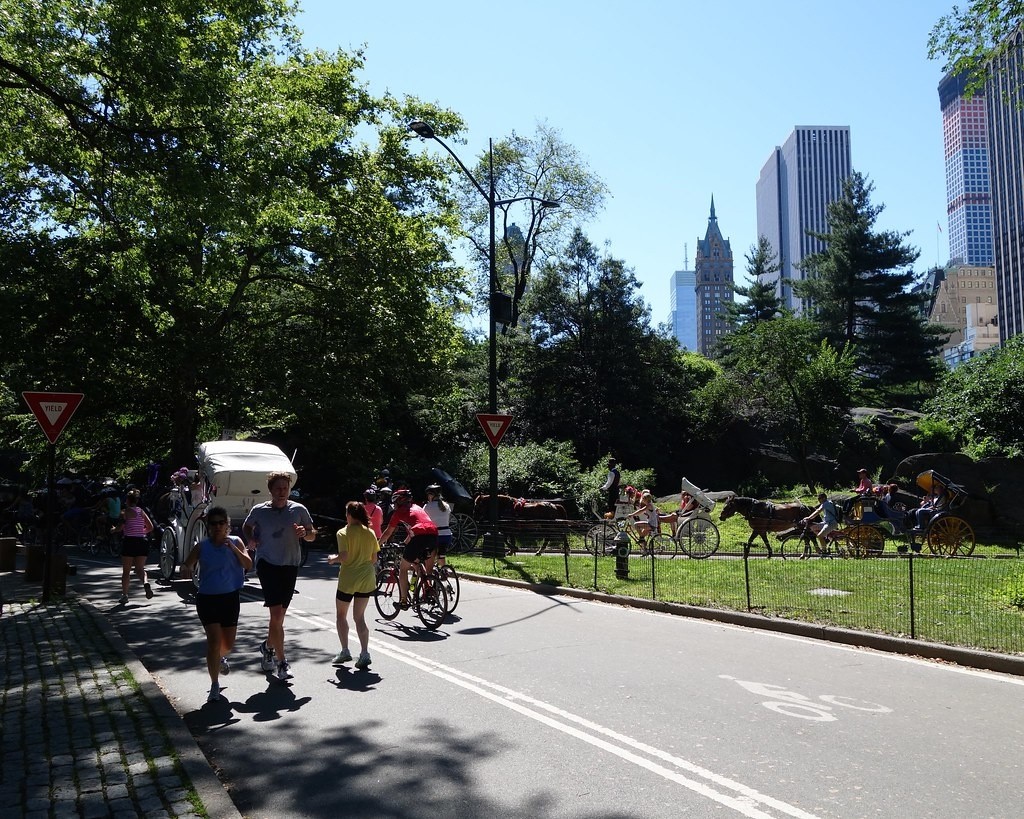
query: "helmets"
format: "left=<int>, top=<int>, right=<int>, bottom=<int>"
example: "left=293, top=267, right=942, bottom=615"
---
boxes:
left=380, top=487, right=392, bottom=495
left=364, top=489, right=376, bottom=498
left=392, top=490, right=413, bottom=506
left=425, top=484, right=442, bottom=494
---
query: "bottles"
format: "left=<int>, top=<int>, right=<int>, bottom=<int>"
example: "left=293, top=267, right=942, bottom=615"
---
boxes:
left=410, top=574, right=417, bottom=592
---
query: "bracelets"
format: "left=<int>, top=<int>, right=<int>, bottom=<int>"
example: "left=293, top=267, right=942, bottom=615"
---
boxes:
left=304, top=531, right=308, bottom=538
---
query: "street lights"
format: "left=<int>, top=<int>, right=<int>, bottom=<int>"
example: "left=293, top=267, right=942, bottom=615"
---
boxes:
left=407, top=121, right=560, bottom=557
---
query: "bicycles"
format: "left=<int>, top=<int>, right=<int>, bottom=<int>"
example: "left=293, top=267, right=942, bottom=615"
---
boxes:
left=781, top=518, right=859, bottom=560
left=603, top=516, right=678, bottom=560
left=77, top=505, right=122, bottom=558
left=375, top=539, right=460, bottom=630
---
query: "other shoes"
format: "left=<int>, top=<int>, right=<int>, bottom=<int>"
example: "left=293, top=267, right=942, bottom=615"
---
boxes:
left=636, top=538, right=644, bottom=544
left=393, top=601, right=408, bottom=611
left=441, top=571, right=449, bottom=581
left=119, top=594, right=129, bottom=603
left=640, top=552, right=649, bottom=557
left=355, top=654, right=371, bottom=667
left=144, top=583, right=153, bottom=599
left=422, top=587, right=435, bottom=598
left=915, top=525, right=926, bottom=529
left=331, top=650, right=351, bottom=663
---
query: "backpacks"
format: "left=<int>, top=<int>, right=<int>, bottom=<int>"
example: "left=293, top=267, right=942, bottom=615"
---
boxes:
left=826, top=503, right=843, bottom=523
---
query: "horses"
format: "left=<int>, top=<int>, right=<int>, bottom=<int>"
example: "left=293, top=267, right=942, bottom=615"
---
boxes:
left=471, top=490, right=572, bottom=557
left=719, top=494, right=824, bottom=560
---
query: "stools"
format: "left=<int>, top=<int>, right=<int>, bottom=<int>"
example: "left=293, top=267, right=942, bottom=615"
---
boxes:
left=859, top=495, right=876, bottom=501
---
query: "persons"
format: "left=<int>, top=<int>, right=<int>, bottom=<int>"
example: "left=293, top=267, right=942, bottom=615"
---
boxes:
left=110, top=489, right=154, bottom=603
left=180, top=507, right=252, bottom=703
left=803, top=492, right=838, bottom=554
left=883, top=484, right=898, bottom=507
left=328, top=482, right=453, bottom=667
left=242, top=471, right=316, bottom=680
left=600, top=458, right=620, bottom=519
left=2, top=475, right=138, bottom=546
left=850, top=468, right=872, bottom=517
left=624, top=486, right=695, bottom=558
left=907, top=484, right=949, bottom=529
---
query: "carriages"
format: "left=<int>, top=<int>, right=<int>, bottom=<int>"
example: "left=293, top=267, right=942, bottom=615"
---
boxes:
left=719, top=469, right=977, bottom=555
left=471, top=473, right=721, bottom=559
left=153, top=440, right=309, bottom=588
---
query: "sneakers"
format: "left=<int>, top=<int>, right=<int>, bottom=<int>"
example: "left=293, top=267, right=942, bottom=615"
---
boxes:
left=208, top=682, right=220, bottom=702
left=220, top=656, right=230, bottom=675
left=260, top=639, right=275, bottom=671
left=276, top=661, right=294, bottom=679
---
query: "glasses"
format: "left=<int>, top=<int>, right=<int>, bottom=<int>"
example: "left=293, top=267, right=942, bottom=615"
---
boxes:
left=208, top=520, right=227, bottom=526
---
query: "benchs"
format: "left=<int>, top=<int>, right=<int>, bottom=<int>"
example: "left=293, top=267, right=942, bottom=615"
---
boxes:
left=881, top=500, right=905, bottom=519
left=676, top=508, right=699, bottom=526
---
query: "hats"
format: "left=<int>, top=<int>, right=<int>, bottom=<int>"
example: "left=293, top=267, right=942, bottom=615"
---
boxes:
left=857, top=469, right=867, bottom=473
